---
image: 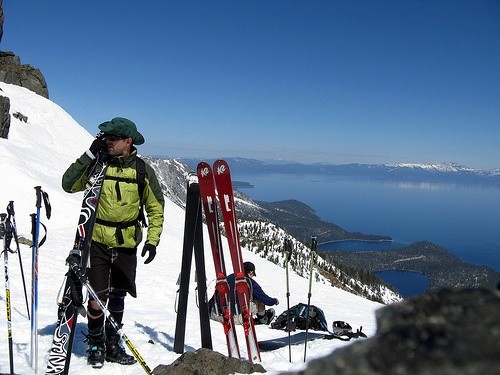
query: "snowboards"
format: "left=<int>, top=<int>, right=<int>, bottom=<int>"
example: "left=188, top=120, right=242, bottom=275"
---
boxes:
left=45, top=157, right=108, bottom=375
left=257, top=331, right=326, bottom=351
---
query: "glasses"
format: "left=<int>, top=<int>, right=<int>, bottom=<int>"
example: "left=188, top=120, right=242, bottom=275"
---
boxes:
left=105, top=133, right=129, bottom=141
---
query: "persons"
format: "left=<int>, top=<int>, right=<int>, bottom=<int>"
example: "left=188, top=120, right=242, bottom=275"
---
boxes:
left=62, top=117, right=165, bottom=369
left=211, top=262, right=279, bottom=325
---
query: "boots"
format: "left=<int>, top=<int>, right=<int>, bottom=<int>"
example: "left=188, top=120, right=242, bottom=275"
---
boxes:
left=104, top=321, right=137, bottom=365
left=81, top=327, right=105, bottom=369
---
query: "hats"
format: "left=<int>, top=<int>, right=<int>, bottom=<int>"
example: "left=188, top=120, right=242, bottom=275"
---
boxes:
left=98, top=117, right=145, bottom=145
left=244, top=261, right=256, bottom=277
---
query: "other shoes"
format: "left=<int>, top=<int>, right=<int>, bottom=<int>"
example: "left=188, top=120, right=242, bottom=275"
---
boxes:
left=256, top=308, right=276, bottom=325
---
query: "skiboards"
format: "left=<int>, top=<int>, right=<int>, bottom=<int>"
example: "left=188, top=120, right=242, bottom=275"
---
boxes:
left=197, top=159, right=263, bottom=364
left=173, top=171, right=213, bottom=354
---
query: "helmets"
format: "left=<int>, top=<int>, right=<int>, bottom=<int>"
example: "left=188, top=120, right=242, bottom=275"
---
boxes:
left=332, top=320, right=352, bottom=335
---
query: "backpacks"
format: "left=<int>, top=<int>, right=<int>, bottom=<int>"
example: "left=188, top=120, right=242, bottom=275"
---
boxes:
left=227, top=273, right=252, bottom=325
left=276, top=302, right=328, bottom=331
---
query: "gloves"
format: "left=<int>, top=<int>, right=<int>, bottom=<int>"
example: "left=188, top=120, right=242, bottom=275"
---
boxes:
left=89, top=134, right=109, bottom=156
left=141, top=244, right=157, bottom=264
